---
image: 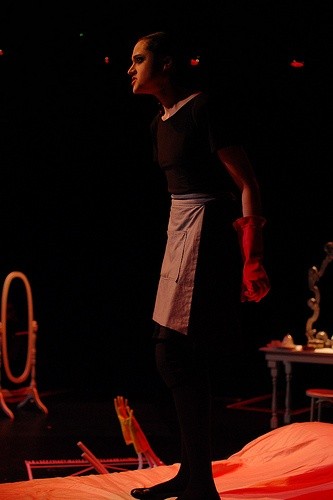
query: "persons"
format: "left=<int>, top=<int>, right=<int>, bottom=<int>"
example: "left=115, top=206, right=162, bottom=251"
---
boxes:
left=127, top=27, right=271, bottom=500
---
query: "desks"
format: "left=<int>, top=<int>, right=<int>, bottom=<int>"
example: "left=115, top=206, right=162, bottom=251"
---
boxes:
left=260, top=340, right=333, bottom=434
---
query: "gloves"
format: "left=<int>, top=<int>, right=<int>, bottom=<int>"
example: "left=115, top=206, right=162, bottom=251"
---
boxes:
left=232, top=214, right=273, bottom=303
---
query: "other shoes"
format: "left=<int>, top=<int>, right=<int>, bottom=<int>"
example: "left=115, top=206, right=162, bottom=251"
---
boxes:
left=131, top=486, right=175, bottom=500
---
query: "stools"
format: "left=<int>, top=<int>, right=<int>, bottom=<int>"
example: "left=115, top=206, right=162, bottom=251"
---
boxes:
left=305, top=386, right=333, bottom=426
